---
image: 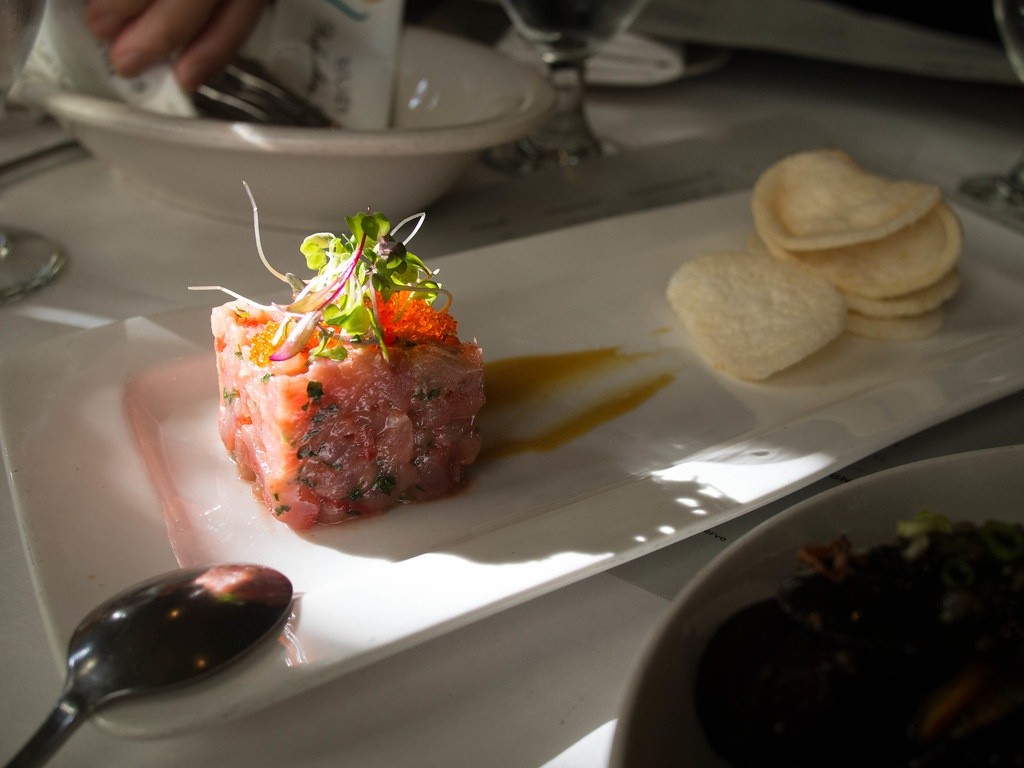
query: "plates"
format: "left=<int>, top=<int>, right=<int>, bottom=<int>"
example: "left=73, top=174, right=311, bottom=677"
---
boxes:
left=0, top=147, right=1024, bottom=735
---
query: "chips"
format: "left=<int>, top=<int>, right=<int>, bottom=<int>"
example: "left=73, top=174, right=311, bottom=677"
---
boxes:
left=663, top=144, right=964, bottom=380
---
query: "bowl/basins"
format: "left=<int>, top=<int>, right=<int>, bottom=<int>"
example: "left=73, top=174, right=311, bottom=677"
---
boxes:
left=601, top=441, right=1024, bottom=768
left=6, top=20, right=566, bottom=242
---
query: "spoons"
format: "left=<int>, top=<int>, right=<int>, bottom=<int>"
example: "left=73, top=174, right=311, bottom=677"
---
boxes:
left=0, top=562, right=294, bottom=768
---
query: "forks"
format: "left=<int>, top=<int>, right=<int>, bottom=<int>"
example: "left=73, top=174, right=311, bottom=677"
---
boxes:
left=165, top=39, right=282, bottom=124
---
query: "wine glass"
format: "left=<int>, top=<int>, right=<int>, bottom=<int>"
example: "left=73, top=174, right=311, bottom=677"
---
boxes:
left=477, top=0, right=653, bottom=176
left=955, top=0, right=1024, bottom=229
left=0, top=0, right=72, bottom=310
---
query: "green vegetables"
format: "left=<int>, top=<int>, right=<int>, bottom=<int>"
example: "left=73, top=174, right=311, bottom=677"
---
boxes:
left=186, top=179, right=454, bottom=370
